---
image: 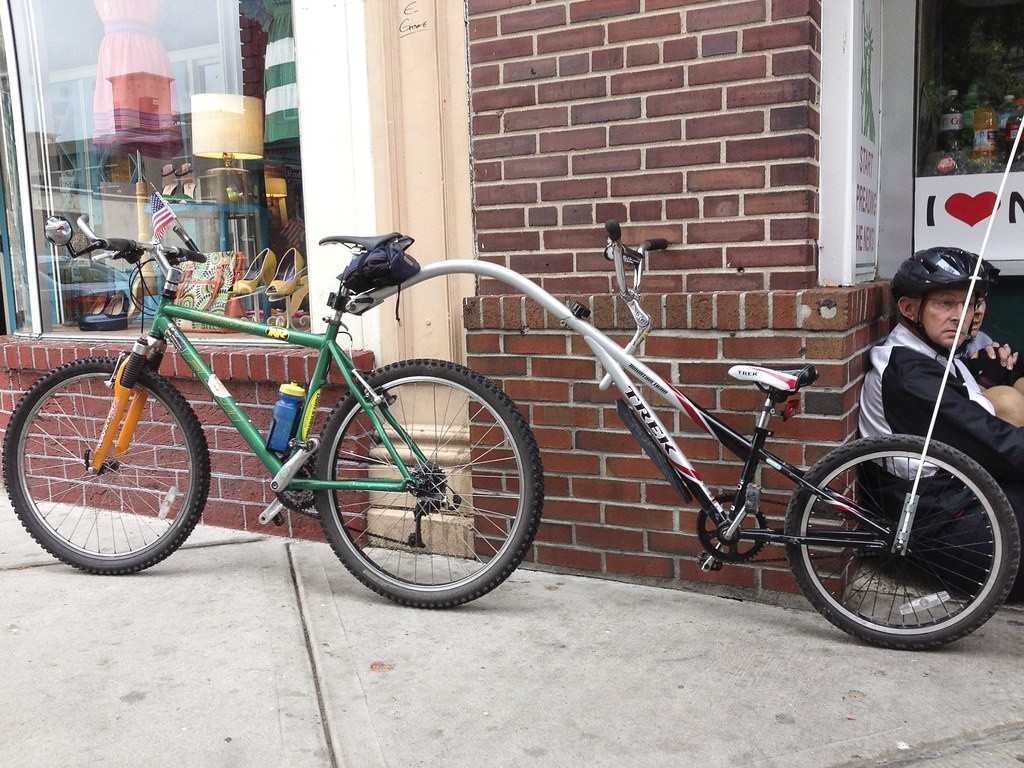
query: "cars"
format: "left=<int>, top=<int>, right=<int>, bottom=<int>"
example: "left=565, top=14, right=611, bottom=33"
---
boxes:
left=26, top=251, right=160, bottom=329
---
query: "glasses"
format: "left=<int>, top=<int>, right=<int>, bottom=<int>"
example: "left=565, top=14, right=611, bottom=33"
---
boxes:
left=919, top=297, right=981, bottom=311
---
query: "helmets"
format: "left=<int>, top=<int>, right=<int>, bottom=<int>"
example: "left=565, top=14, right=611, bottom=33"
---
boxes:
left=892, top=247, right=1001, bottom=287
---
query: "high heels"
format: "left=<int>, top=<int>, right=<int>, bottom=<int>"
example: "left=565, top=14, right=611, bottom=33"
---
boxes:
left=159, top=168, right=197, bottom=203
left=233, top=248, right=304, bottom=296
left=80, top=291, right=128, bottom=331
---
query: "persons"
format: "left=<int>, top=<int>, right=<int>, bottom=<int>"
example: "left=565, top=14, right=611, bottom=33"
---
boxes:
left=89, top=0, right=299, bottom=202
left=950, top=250, right=1024, bottom=428
left=857, top=246, right=1024, bottom=601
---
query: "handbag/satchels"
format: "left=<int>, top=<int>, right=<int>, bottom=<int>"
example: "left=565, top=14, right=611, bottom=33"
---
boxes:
left=171, top=251, right=248, bottom=333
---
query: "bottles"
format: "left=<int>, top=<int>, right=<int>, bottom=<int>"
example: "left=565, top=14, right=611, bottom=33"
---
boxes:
left=998, top=94, right=1016, bottom=162
left=939, top=88, right=963, bottom=151
left=265, top=378, right=305, bottom=454
left=972, top=93, right=996, bottom=158
left=963, top=91, right=981, bottom=147
left=1005, top=97, right=1024, bottom=171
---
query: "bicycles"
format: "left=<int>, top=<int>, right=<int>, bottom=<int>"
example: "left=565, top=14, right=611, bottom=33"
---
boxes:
left=1, top=180, right=1024, bottom=650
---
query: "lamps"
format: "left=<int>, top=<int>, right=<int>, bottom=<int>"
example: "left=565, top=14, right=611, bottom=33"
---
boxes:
left=190, top=92, right=265, bottom=201
